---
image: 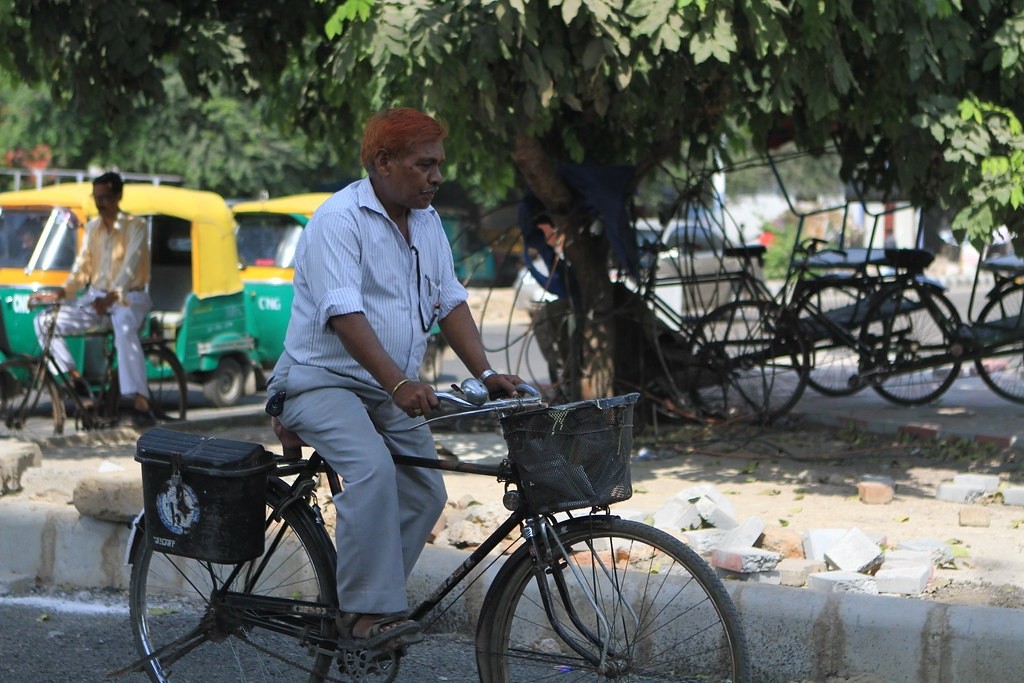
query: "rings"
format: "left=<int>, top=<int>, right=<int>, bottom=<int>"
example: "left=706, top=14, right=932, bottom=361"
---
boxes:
left=413, top=408, right=422, bottom=417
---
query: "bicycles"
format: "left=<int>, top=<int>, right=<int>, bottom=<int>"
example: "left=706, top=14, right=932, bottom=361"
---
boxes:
left=0, top=299, right=189, bottom=442
left=105, top=377, right=755, bottom=683
left=681, top=237, right=1024, bottom=427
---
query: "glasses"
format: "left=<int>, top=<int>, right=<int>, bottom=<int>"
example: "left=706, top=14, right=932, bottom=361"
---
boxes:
left=89, top=193, right=118, bottom=201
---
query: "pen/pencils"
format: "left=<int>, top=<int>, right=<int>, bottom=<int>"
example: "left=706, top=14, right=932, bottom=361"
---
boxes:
left=425, top=273, right=433, bottom=297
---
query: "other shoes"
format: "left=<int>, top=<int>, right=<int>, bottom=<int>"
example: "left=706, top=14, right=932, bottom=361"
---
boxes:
left=132, top=406, right=157, bottom=429
left=60, top=376, right=89, bottom=397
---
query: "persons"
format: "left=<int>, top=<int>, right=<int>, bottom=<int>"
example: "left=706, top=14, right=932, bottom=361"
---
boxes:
left=19, top=220, right=75, bottom=272
left=30, top=171, right=156, bottom=430
left=266, top=108, right=529, bottom=666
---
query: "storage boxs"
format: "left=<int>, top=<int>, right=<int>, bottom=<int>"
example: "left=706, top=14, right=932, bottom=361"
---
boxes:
left=133, top=429, right=266, bottom=566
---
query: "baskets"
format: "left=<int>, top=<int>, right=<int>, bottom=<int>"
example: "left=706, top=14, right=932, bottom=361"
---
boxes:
left=498, top=393, right=641, bottom=514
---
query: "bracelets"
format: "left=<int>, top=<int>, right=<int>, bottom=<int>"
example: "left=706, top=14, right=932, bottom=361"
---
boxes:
left=477, top=369, right=499, bottom=383
left=391, top=378, right=411, bottom=401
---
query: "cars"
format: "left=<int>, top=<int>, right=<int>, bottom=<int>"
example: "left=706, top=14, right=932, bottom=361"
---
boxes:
left=510, top=215, right=764, bottom=323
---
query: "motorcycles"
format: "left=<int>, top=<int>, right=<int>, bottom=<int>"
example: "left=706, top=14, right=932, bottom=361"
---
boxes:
left=230, top=191, right=451, bottom=383
left=0, top=183, right=266, bottom=408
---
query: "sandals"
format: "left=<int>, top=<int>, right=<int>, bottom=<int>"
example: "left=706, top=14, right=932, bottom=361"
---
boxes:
left=335, top=609, right=422, bottom=650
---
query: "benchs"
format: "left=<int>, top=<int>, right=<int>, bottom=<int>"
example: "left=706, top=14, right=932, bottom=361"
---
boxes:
left=149, top=264, right=193, bottom=329
